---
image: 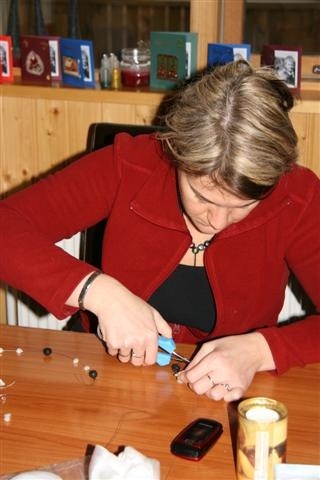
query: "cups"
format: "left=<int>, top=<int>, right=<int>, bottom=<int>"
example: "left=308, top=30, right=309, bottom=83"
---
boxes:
left=235, top=396, right=288, bottom=480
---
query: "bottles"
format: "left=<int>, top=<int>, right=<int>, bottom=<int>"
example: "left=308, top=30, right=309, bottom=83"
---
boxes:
left=121, top=47, right=152, bottom=86
left=100, top=52, right=122, bottom=89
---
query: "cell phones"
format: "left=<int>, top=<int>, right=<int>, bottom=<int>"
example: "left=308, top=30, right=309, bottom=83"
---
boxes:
left=170, top=417, right=224, bottom=463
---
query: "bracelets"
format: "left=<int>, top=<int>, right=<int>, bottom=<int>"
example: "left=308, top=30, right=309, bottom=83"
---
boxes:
left=78, top=271, right=104, bottom=310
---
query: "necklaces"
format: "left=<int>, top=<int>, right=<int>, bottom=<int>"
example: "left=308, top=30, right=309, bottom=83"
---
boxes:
left=189, top=240, right=210, bottom=266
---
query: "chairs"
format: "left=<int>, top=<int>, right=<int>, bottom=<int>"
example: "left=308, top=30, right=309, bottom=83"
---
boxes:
left=78, top=123, right=171, bottom=269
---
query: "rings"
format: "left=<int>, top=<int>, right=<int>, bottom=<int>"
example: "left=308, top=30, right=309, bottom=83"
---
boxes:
left=119, top=353, right=130, bottom=357
left=221, top=384, right=231, bottom=391
left=132, top=352, right=144, bottom=358
left=207, top=375, right=216, bottom=386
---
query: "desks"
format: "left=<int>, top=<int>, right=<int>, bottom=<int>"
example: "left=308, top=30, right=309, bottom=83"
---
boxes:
left=0, top=323, right=320, bottom=480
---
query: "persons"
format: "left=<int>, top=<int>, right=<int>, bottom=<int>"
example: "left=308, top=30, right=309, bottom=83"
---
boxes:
left=0, top=59, right=320, bottom=402
left=283, top=56, right=295, bottom=85
